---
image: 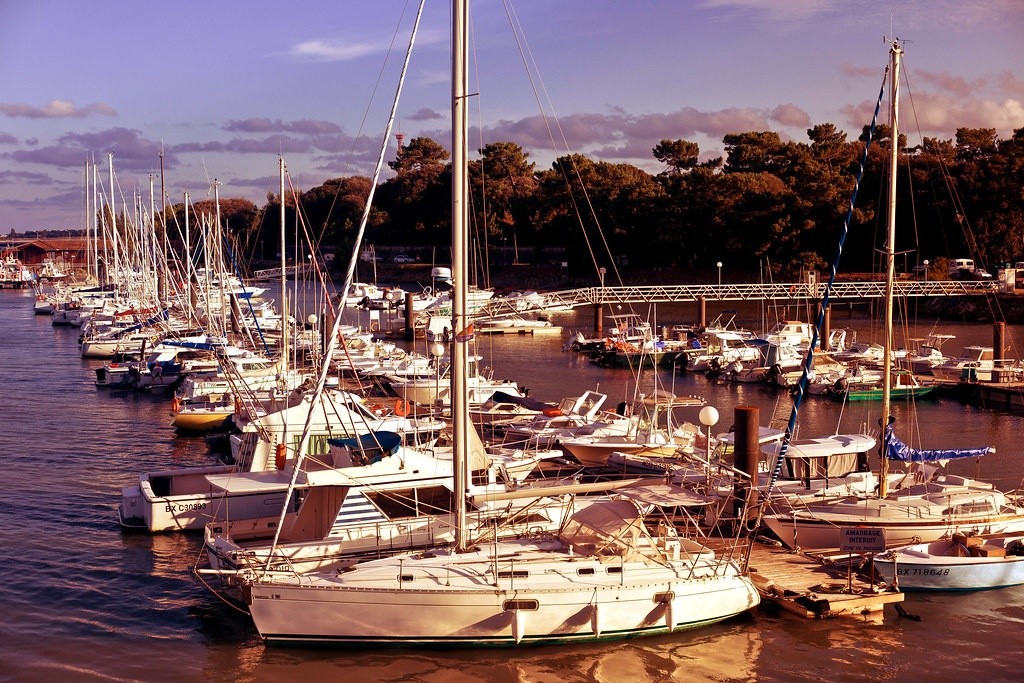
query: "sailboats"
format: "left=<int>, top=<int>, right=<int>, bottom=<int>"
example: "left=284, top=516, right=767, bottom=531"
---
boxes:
left=0, top=1, right=1021, bottom=649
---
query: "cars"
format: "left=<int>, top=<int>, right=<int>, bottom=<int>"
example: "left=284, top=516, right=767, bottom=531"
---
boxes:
left=911, top=264, right=923, bottom=272
left=359, top=253, right=382, bottom=263
left=975, top=269, right=993, bottom=278
left=394, top=254, right=415, bottom=264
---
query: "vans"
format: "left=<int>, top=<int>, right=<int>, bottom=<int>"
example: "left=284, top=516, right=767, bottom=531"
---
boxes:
left=947, top=259, right=975, bottom=277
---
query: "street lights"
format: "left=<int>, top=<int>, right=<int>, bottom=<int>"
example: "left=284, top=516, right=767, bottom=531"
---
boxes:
left=503, top=238, right=508, bottom=266
left=716, top=262, right=722, bottom=300
left=923, top=260, right=929, bottom=282
left=260, top=241, right=264, bottom=263
